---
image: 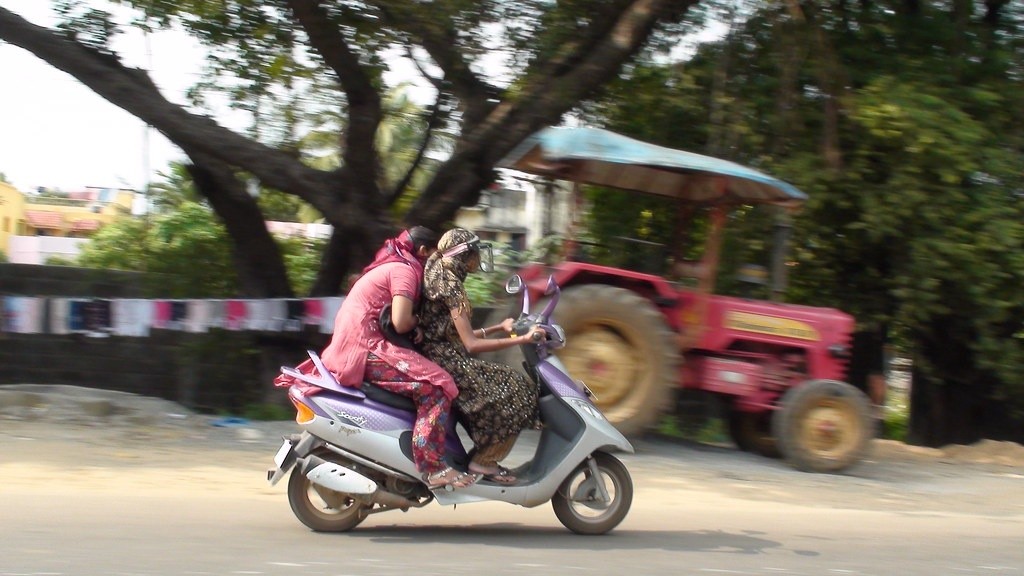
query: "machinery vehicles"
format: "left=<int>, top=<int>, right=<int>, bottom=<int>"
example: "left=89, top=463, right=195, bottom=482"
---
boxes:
left=475, top=124, right=874, bottom=476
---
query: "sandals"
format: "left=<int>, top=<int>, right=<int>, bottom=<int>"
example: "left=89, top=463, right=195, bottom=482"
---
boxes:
left=468, top=464, right=521, bottom=486
left=427, top=466, right=484, bottom=490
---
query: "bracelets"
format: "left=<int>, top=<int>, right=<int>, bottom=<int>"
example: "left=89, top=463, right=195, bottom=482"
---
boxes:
left=480, top=328, right=487, bottom=338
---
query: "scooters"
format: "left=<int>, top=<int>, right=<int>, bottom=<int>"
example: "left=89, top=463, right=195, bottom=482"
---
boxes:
left=266, top=274, right=636, bottom=535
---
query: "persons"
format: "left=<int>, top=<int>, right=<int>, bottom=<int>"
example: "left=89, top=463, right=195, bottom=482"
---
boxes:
left=323, top=226, right=484, bottom=490
left=417, top=227, right=546, bottom=484
